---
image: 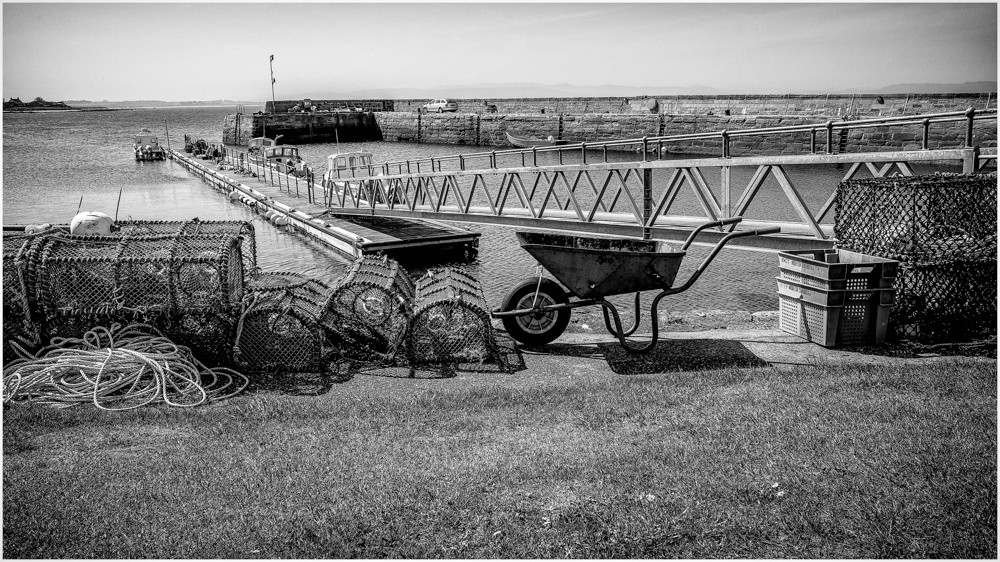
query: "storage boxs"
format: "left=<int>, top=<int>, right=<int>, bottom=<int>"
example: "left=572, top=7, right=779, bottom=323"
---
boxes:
left=775, top=248, right=901, bottom=348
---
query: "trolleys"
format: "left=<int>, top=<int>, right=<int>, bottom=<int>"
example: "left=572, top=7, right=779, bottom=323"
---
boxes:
left=490, top=214, right=782, bottom=354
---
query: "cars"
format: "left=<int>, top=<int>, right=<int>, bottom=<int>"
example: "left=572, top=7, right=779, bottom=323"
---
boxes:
left=422, top=98, right=459, bottom=113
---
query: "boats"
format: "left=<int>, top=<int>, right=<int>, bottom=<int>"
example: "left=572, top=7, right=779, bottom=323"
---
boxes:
left=264, top=145, right=314, bottom=180
left=133, top=128, right=167, bottom=162
left=247, top=137, right=275, bottom=166
left=321, top=151, right=402, bottom=203
